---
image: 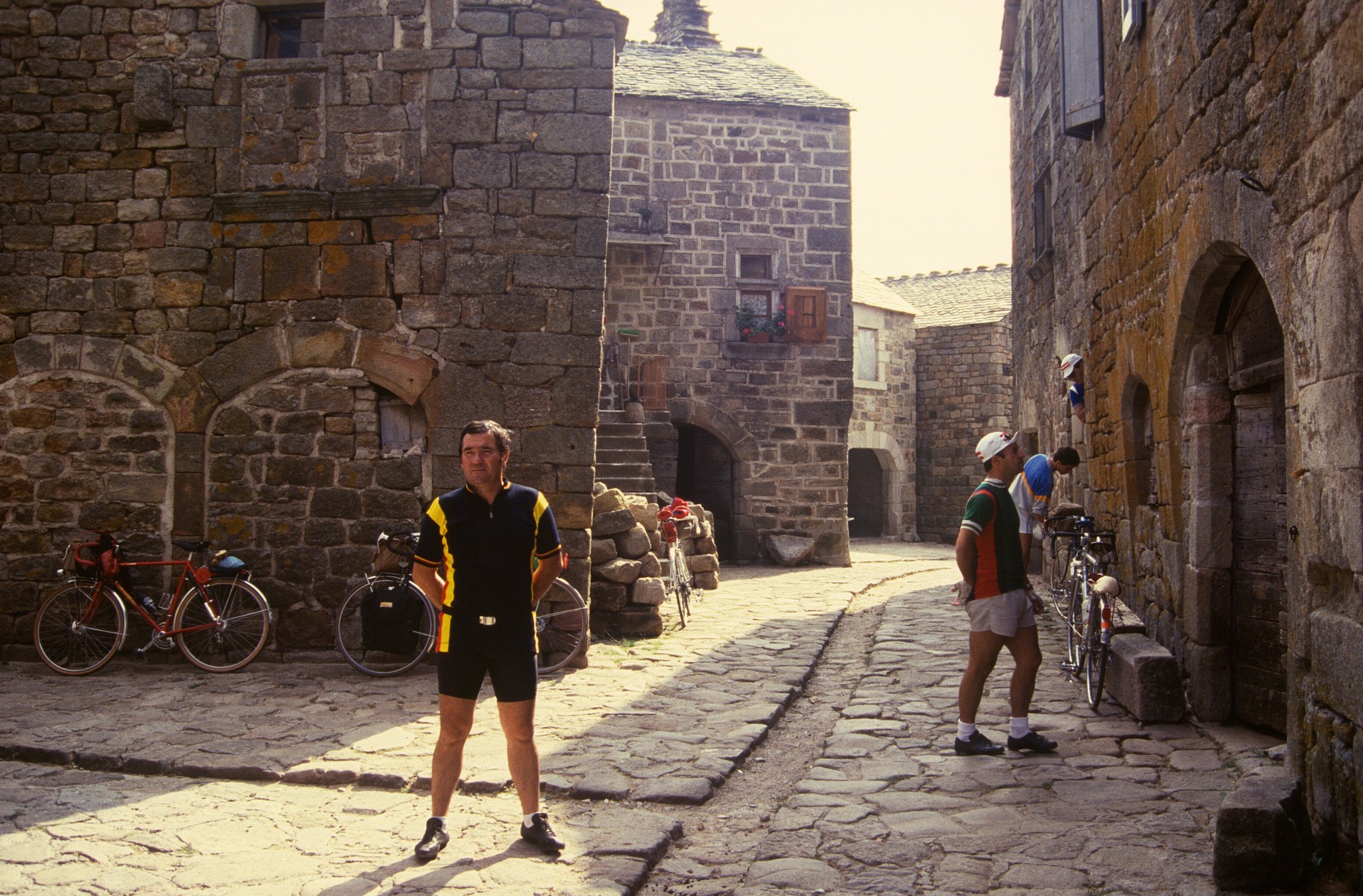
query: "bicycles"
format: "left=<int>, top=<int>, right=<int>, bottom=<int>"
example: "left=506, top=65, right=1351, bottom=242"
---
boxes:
left=1043, top=516, right=1121, bottom=709
left=32, top=527, right=270, bottom=677
left=660, top=515, right=701, bottom=629
left=336, top=531, right=586, bottom=677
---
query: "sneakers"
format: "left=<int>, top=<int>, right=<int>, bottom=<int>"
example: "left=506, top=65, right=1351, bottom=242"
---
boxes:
left=520, top=812, right=565, bottom=851
left=953, top=729, right=1004, bottom=756
left=414, top=818, right=450, bottom=858
left=1006, top=731, right=1058, bottom=751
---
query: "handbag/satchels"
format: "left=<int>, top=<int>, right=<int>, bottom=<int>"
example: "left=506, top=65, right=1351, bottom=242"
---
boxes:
left=370, top=536, right=411, bottom=573
left=1053, top=503, right=1085, bottom=537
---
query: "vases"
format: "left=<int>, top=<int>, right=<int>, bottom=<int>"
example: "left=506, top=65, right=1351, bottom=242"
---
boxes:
left=744, top=332, right=770, bottom=343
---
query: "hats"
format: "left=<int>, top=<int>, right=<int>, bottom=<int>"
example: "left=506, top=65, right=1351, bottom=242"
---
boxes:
left=1061, top=354, right=1082, bottom=378
left=975, top=431, right=1019, bottom=462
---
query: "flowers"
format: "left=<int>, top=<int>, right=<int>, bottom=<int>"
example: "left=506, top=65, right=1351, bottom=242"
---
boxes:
left=730, top=302, right=794, bottom=336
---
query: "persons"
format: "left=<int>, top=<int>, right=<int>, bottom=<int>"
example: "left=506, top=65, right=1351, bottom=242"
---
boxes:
left=1059, top=352, right=1084, bottom=424
left=409, top=416, right=568, bottom=862
left=1007, top=446, right=1081, bottom=587
left=948, top=431, right=1063, bottom=756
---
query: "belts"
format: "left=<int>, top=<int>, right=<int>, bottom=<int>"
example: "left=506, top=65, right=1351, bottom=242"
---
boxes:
left=441, top=604, right=532, bottom=625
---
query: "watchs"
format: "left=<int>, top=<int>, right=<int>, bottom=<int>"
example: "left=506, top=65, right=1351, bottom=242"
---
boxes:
left=1023, top=582, right=1032, bottom=590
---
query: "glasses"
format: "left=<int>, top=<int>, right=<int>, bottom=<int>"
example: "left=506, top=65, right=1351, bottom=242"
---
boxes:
left=1066, top=367, right=1081, bottom=382
